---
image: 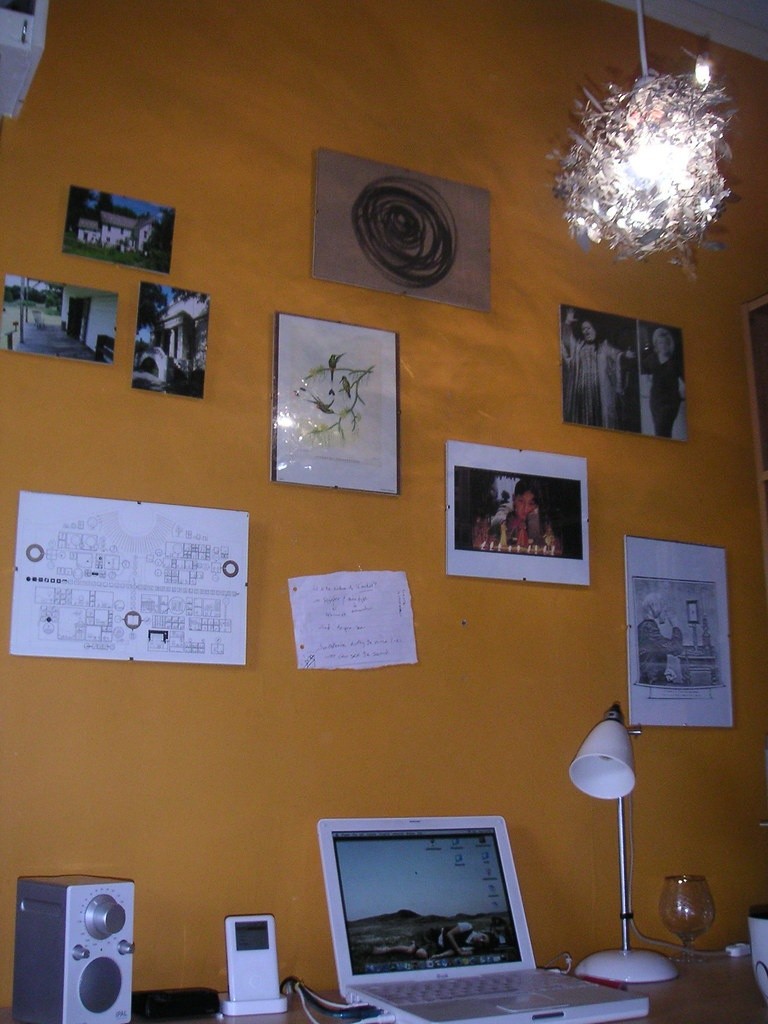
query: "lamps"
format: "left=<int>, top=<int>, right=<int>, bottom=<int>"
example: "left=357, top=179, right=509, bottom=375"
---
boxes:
left=568, top=701, right=679, bottom=983
left=545, top=0, right=743, bottom=284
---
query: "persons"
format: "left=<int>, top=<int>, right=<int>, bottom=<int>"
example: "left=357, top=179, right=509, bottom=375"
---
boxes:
left=371, top=921, right=501, bottom=960
left=496, top=478, right=545, bottom=549
left=642, top=327, right=683, bottom=438
left=562, top=308, right=637, bottom=428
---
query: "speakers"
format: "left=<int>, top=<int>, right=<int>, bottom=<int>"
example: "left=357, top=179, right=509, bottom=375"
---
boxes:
left=13, top=873, right=132, bottom=1023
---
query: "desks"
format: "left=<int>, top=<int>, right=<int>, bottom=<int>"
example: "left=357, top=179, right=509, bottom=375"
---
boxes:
left=0, top=953, right=768, bottom=1024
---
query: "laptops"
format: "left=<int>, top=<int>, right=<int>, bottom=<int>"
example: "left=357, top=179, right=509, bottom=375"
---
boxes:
left=317, top=816, right=649, bottom=1024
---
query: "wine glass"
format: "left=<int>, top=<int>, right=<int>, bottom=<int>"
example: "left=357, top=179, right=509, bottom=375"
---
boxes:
left=661, top=873, right=714, bottom=964
